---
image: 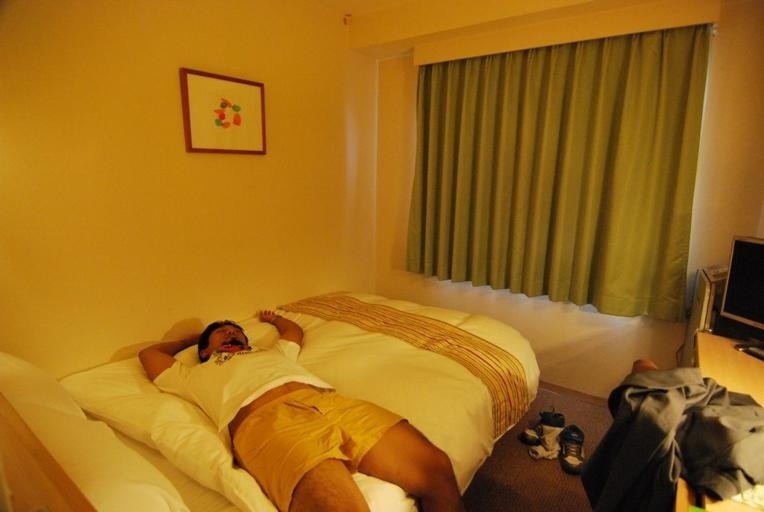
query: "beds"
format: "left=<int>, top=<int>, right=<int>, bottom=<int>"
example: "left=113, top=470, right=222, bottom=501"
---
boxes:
left=0, top=293, right=541, bottom=512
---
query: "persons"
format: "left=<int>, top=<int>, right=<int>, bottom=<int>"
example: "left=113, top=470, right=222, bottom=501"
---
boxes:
left=139, top=310, right=468, bottom=512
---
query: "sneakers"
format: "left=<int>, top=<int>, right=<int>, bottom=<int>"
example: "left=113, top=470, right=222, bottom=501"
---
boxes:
left=561, top=425, right=584, bottom=474
left=519, top=412, right=565, bottom=445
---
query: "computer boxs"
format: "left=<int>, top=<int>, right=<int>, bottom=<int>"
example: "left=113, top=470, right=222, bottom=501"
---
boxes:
left=679, top=263, right=729, bottom=367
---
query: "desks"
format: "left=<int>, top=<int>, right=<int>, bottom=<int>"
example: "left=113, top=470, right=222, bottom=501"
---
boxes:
left=674, top=332, right=764, bottom=512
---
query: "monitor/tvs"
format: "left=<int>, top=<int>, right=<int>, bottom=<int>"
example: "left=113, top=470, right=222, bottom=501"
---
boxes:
left=720, top=235, right=764, bottom=361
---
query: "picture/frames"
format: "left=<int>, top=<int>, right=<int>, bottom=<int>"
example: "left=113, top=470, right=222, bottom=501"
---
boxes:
left=179, top=67, right=267, bottom=155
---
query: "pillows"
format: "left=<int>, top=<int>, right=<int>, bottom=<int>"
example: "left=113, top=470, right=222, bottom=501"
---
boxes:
left=0, top=350, right=191, bottom=512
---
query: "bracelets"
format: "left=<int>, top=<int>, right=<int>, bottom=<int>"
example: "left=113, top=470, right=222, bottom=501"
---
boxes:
left=270, top=315, right=281, bottom=325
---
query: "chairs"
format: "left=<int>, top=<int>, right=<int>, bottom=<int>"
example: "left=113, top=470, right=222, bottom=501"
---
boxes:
left=633, top=358, right=658, bottom=375
